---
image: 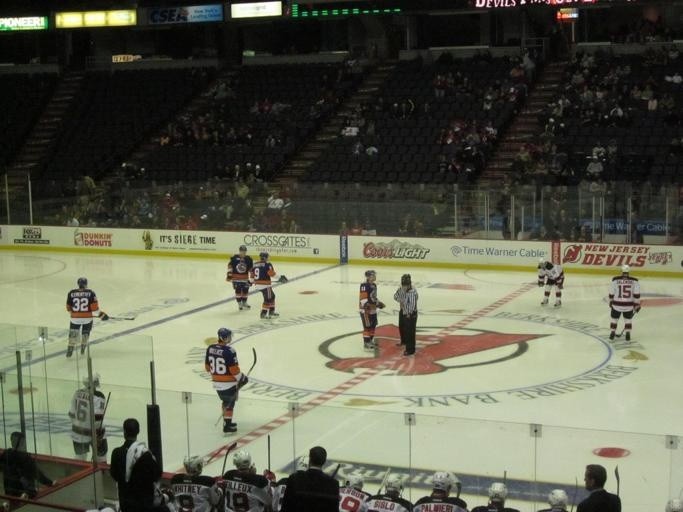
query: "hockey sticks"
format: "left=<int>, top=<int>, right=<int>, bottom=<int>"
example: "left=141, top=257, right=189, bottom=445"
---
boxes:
left=456, top=482, right=462, bottom=499
left=214, top=347, right=256, bottom=426
left=222, top=442, right=237, bottom=475
left=615, top=300, right=644, bottom=337
left=614, top=466, right=619, bottom=496
left=91, top=315, right=134, bottom=320
left=569, top=476, right=578, bottom=512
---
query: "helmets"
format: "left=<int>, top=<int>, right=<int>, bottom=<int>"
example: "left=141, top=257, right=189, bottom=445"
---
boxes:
left=78, top=277, right=87, bottom=288
left=233, top=450, right=250, bottom=469
left=538, top=257, right=547, bottom=265
left=239, top=245, right=247, bottom=250
left=82, top=372, right=100, bottom=388
left=385, top=473, right=402, bottom=495
left=433, top=472, right=452, bottom=497
left=217, top=328, right=231, bottom=339
left=401, top=274, right=411, bottom=286
left=666, top=498, right=683, bottom=512
left=622, top=265, right=630, bottom=272
left=260, top=252, right=268, bottom=260
left=365, top=270, right=375, bottom=276
left=489, top=483, right=507, bottom=501
left=183, top=453, right=202, bottom=474
left=296, top=457, right=309, bottom=471
left=345, top=473, right=362, bottom=490
left=547, top=489, right=567, bottom=506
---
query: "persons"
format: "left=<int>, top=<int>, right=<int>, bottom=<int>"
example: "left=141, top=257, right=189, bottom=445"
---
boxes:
left=226, top=245, right=255, bottom=310
left=68, top=370, right=108, bottom=464
left=66, top=278, right=109, bottom=358
left=394, top=274, right=419, bottom=355
left=608, top=264, right=641, bottom=341
left=205, top=327, right=257, bottom=433
left=1, top=13, right=682, bottom=245
left=252, top=251, right=288, bottom=319
left=0, top=432, right=57, bottom=510
left=359, top=269, right=386, bottom=348
left=86, top=419, right=621, bottom=512
left=537, top=261, right=565, bottom=308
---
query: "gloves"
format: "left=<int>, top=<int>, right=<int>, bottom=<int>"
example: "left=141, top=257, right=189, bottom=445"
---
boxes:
left=376, top=302, right=385, bottom=309
left=558, top=285, right=563, bottom=288
left=538, top=284, right=543, bottom=287
left=239, top=373, right=248, bottom=384
left=98, top=311, right=109, bottom=320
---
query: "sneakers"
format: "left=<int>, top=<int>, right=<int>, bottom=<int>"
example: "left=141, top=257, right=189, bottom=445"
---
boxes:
left=625, top=332, right=630, bottom=341
left=403, top=350, right=414, bottom=356
left=364, top=336, right=378, bottom=349
left=238, top=302, right=242, bottom=309
left=260, top=309, right=270, bottom=319
left=269, top=307, right=279, bottom=316
left=66, top=347, right=73, bottom=357
left=224, top=418, right=237, bottom=432
left=81, top=344, right=87, bottom=353
left=554, top=301, right=560, bottom=306
left=541, top=299, right=548, bottom=304
left=372, top=337, right=378, bottom=345
left=231, top=423, right=237, bottom=426
left=395, top=343, right=404, bottom=347
left=609, top=331, right=614, bottom=340
left=243, top=303, right=251, bottom=308
